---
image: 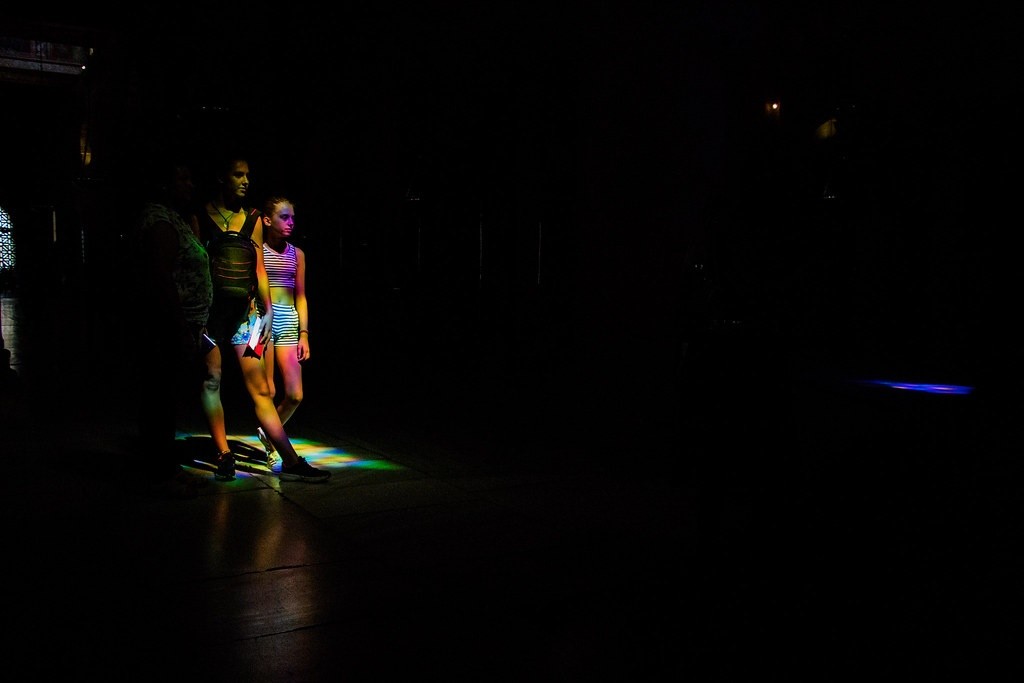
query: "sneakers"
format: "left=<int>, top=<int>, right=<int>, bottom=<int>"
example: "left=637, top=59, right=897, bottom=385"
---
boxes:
left=214, top=452, right=236, bottom=480
left=279, top=456, right=332, bottom=481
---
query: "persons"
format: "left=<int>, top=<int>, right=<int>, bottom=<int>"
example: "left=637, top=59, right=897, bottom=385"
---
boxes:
left=253, top=196, right=311, bottom=473
left=176, top=150, right=331, bottom=481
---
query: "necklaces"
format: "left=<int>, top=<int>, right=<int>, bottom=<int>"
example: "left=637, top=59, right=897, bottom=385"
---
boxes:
left=212, top=200, right=234, bottom=229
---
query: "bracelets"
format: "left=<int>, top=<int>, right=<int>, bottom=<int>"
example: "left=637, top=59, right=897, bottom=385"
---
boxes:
left=299, top=329, right=309, bottom=334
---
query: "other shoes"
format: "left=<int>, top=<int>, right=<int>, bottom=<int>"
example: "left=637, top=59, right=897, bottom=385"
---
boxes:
left=257, top=427, right=282, bottom=472
